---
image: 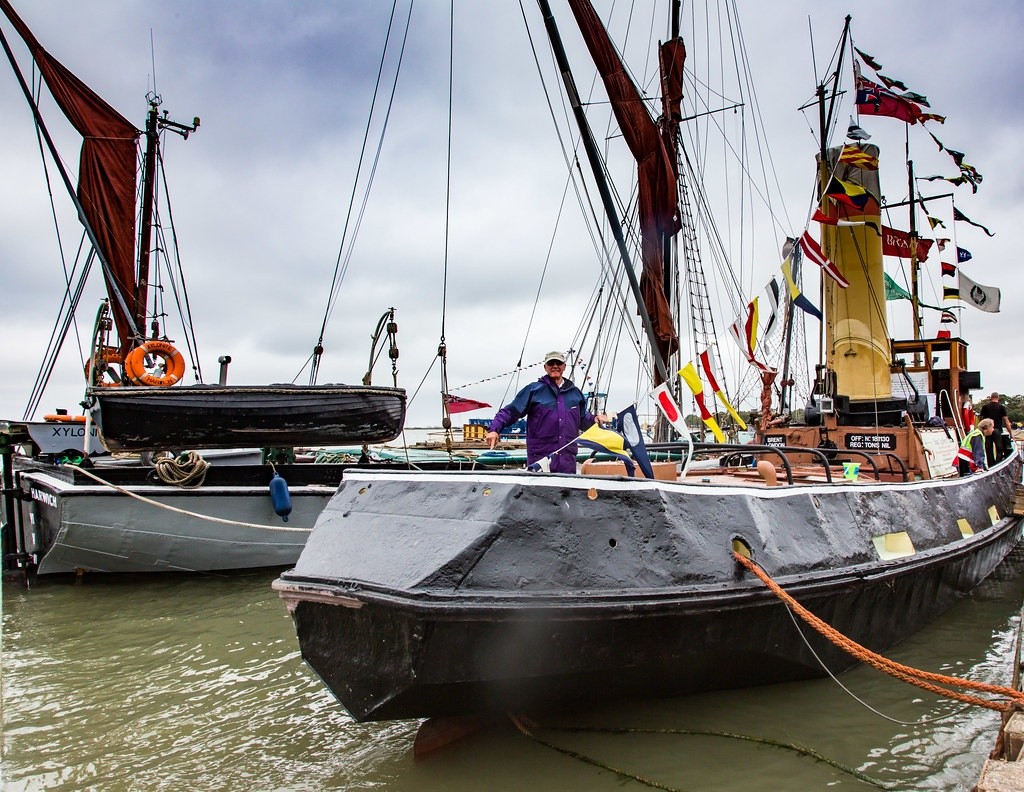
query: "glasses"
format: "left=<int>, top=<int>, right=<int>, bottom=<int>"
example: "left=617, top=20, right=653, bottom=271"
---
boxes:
left=547, top=361, right=562, bottom=366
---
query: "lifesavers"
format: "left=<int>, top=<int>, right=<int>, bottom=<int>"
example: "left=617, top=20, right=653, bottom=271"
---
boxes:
left=125, top=346, right=174, bottom=386
left=43, top=414, right=86, bottom=421
left=132, top=341, right=185, bottom=385
left=84, top=348, right=124, bottom=387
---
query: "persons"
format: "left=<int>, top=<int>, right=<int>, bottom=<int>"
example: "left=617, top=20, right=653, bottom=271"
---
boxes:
left=951, top=418, right=994, bottom=477
left=486, top=352, right=608, bottom=475
left=980, top=392, right=1014, bottom=468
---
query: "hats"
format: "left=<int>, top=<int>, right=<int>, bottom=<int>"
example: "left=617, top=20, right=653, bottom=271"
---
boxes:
left=545, top=351, right=564, bottom=364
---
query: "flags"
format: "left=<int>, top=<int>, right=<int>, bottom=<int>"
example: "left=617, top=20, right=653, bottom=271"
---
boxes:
left=527, top=47, right=1000, bottom=482
left=444, top=393, right=492, bottom=414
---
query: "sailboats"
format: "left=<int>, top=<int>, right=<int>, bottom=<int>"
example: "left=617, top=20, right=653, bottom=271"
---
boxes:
left=0, top=0, right=694, bottom=585
left=269, top=0, right=1024, bottom=726
